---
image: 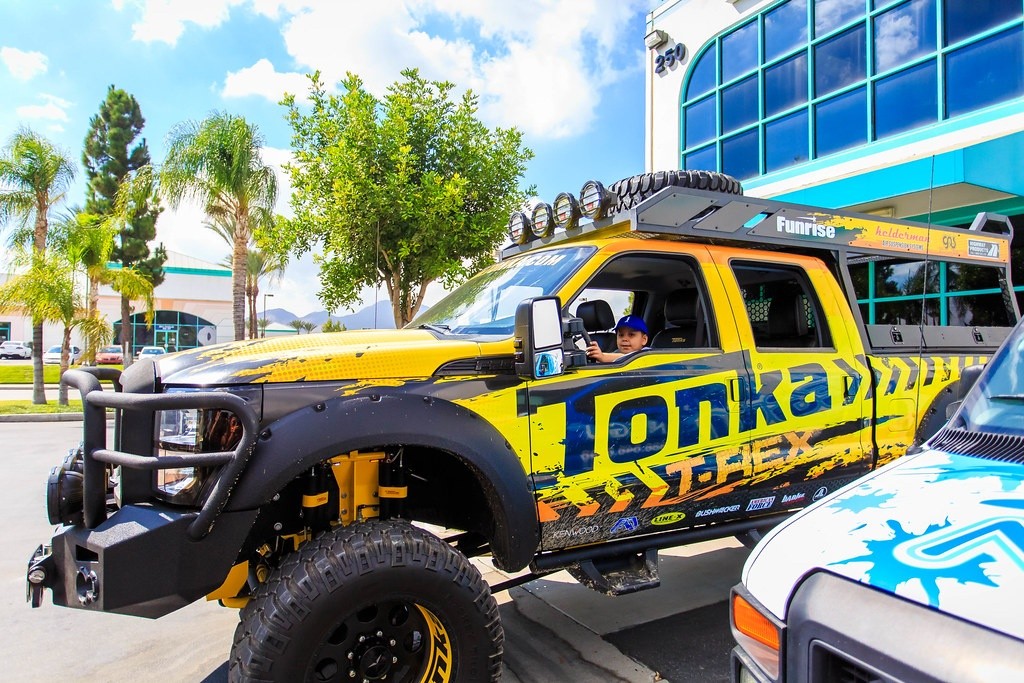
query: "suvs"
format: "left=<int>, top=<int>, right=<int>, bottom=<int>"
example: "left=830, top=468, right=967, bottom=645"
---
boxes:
left=25, top=171, right=1023, bottom=683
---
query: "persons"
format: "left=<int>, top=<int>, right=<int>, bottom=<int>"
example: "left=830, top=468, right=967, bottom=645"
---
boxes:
left=586, top=315, right=653, bottom=364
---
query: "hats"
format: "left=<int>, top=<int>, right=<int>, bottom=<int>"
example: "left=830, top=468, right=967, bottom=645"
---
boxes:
left=612, top=315, right=649, bottom=335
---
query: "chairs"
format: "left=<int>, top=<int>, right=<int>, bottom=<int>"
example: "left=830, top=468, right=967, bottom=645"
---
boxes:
left=756, top=291, right=818, bottom=346
left=576, top=301, right=617, bottom=353
left=650, top=287, right=708, bottom=348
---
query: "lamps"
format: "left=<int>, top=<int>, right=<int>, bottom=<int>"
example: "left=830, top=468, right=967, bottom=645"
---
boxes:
left=643, top=29, right=668, bottom=50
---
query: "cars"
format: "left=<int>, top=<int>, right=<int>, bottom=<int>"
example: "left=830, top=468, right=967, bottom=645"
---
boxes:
left=95, top=344, right=124, bottom=366
left=136, top=346, right=166, bottom=364
left=0, top=341, right=32, bottom=360
left=42, top=345, right=82, bottom=366
left=729, top=308, right=1024, bottom=683
